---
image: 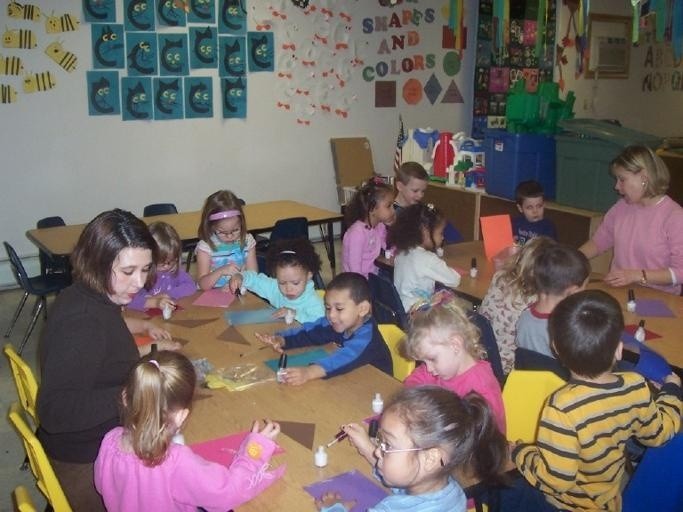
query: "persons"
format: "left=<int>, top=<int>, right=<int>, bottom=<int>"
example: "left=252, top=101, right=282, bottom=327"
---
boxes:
left=576, top=144, right=683, bottom=297
left=195, top=189, right=259, bottom=295
left=122, top=219, right=198, bottom=315
left=340, top=176, right=409, bottom=331
left=91, top=348, right=283, bottom=512
left=514, top=244, right=594, bottom=361
left=35, top=208, right=161, bottom=512
left=228, top=237, right=326, bottom=325
left=391, top=200, right=461, bottom=322
left=401, top=300, right=507, bottom=438
left=512, top=180, right=558, bottom=245
left=253, top=271, right=394, bottom=387
left=478, top=288, right=683, bottom=512
left=314, top=384, right=513, bottom=511
left=476, top=235, right=559, bottom=377
left=379, top=161, right=465, bottom=258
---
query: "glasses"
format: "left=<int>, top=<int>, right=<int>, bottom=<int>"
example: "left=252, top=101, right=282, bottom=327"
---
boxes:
left=215, top=229, right=240, bottom=236
left=156, top=257, right=178, bottom=267
left=374, top=432, right=443, bottom=466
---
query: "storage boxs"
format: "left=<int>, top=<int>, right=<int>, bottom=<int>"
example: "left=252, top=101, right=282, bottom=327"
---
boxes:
left=555, top=118, right=664, bottom=216
left=481, top=128, right=592, bottom=200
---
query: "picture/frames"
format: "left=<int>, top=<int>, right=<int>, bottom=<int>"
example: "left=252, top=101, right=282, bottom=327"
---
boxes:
left=584, top=12, right=633, bottom=79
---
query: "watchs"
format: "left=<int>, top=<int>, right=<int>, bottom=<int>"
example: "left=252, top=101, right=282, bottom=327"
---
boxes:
left=641, top=268, right=648, bottom=284
